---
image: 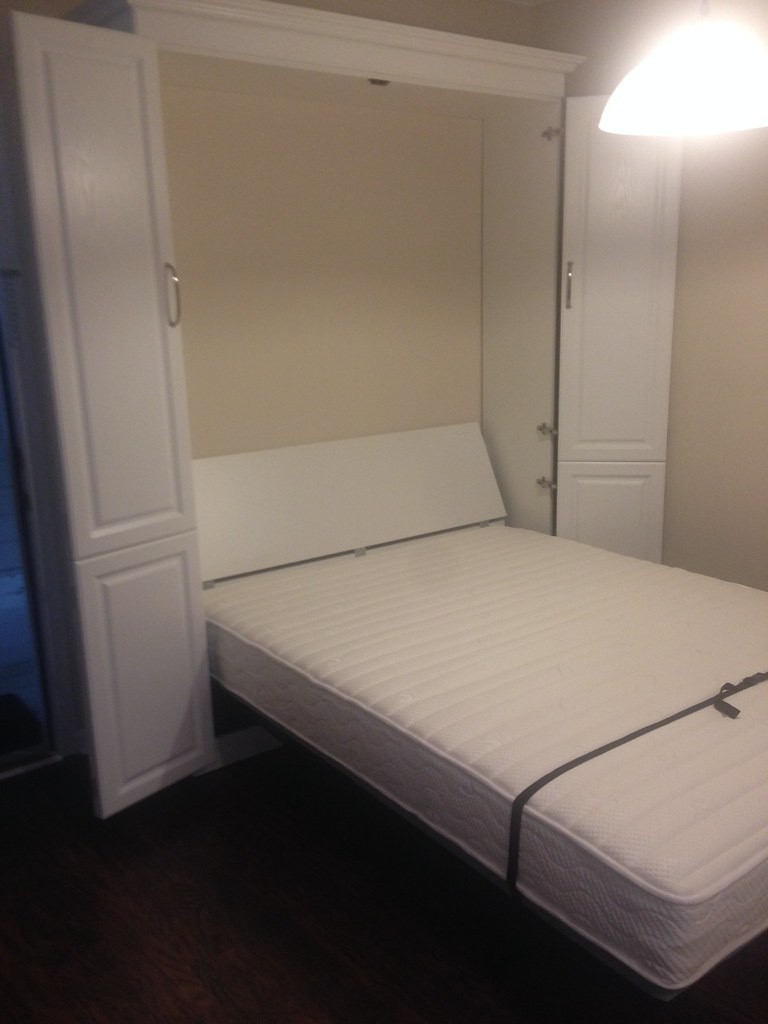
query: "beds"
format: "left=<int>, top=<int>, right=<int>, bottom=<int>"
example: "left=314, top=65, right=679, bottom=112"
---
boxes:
left=189, top=423, right=768, bottom=1001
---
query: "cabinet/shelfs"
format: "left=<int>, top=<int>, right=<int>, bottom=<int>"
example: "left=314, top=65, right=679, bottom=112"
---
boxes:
left=0, top=10, right=220, bottom=822
left=481, top=94, right=682, bottom=564
